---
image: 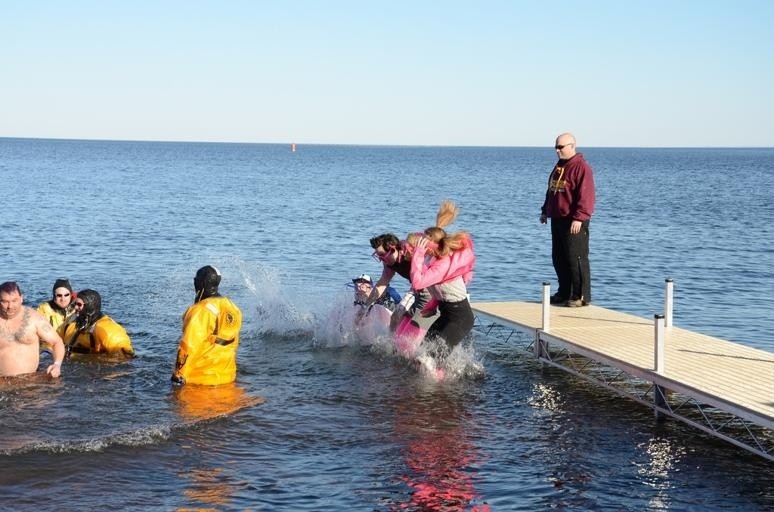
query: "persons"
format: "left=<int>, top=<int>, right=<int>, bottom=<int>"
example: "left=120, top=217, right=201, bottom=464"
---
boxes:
left=170, top=265, right=242, bottom=387
left=408, top=227, right=474, bottom=373
left=35, top=279, right=78, bottom=348
left=540, top=133, right=595, bottom=308
left=354, top=235, right=440, bottom=353
left=66, top=289, right=133, bottom=359
left=0, top=283, right=65, bottom=382
left=353, top=274, right=391, bottom=339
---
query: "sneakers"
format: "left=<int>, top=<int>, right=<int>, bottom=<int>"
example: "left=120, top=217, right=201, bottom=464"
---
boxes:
left=550, top=292, right=568, bottom=306
left=565, top=296, right=588, bottom=308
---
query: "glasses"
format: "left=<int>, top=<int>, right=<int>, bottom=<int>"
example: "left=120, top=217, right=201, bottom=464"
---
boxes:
left=56, top=293, right=71, bottom=298
left=371, top=249, right=392, bottom=265
left=74, top=301, right=86, bottom=307
left=554, top=145, right=573, bottom=150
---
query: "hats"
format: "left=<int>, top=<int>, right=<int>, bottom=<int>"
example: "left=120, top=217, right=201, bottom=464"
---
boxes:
left=352, top=273, right=373, bottom=285
left=53, top=278, right=73, bottom=292
